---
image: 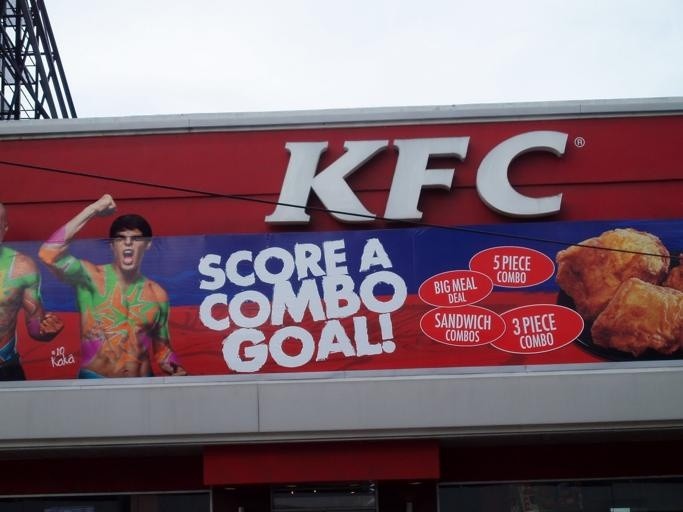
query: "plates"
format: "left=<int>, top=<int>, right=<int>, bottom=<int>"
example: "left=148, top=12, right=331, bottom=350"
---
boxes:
left=555, top=253, right=683, bottom=362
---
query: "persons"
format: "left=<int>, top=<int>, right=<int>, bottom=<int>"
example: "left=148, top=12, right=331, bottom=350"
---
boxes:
left=38, top=193, right=187, bottom=379
left=0, top=204, right=65, bottom=381
